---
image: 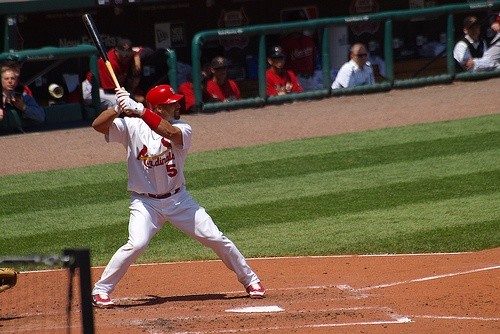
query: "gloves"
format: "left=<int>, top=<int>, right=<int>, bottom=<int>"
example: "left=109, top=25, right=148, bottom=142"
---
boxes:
left=114, top=87, right=144, bottom=116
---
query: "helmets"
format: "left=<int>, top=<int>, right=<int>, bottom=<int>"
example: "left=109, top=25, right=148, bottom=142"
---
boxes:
left=146, top=85, right=184, bottom=112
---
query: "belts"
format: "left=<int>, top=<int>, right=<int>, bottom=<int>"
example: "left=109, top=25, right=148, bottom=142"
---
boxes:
left=134, top=188, right=181, bottom=200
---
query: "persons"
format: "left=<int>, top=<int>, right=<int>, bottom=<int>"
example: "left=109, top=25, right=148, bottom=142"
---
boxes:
left=0, top=34, right=159, bottom=136
left=176, top=44, right=304, bottom=115
left=277, top=10, right=500, bottom=91
left=91, top=84, right=265, bottom=308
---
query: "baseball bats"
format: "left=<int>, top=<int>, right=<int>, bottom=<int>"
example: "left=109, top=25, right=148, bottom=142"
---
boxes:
left=82, top=13, right=132, bottom=115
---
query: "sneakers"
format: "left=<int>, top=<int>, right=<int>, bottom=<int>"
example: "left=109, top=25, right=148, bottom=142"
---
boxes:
left=91, top=294, right=114, bottom=309
left=246, top=281, right=268, bottom=299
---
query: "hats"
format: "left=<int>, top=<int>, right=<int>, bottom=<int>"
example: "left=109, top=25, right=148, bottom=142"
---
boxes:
left=116, top=40, right=133, bottom=56
left=463, top=17, right=481, bottom=29
left=209, top=57, right=232, bottom=72
left=267, top=46, right=285, bottom=59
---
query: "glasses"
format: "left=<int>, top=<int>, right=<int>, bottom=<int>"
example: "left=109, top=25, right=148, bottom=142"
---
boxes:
left=352, top=54, right=367, bottom=58
